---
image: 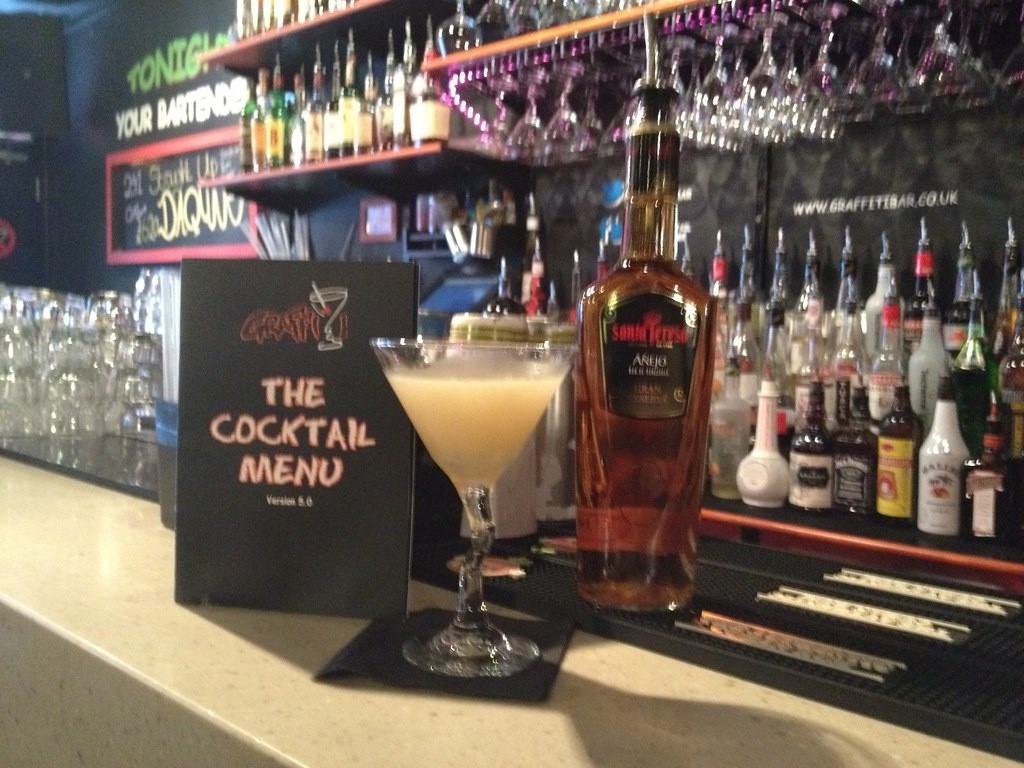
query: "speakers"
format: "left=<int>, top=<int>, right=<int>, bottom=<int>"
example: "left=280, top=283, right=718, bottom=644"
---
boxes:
left=0, top=10, right=71, bottom=136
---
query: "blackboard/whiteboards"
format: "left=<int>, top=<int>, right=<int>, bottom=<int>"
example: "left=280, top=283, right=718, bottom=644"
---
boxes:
left=105, top=123, right=261, bottom=264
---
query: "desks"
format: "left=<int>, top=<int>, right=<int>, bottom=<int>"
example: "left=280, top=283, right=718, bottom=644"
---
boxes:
left=0, top=426, right=1024, bottom=768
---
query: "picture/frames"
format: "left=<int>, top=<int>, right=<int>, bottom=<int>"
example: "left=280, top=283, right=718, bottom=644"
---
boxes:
left=358, top=198, right=397, bottom=244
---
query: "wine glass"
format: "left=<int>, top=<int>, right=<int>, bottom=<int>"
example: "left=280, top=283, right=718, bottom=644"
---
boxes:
left=437, top=0, right=1024, bottom=166
left=371, top=338, right=574, bottom=677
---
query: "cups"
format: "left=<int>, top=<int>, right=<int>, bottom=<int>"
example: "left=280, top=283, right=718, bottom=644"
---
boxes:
left=0, top=283, right=165, bottom=444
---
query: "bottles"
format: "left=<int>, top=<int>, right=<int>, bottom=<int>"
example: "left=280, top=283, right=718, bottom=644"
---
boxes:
left=569, top=13, right=718, bottom=616
left=673, top=216, right=1024, bottom=544
left=235, top=0, right=450, bottom=174
left=446, top=222, right=623, bottom=542
